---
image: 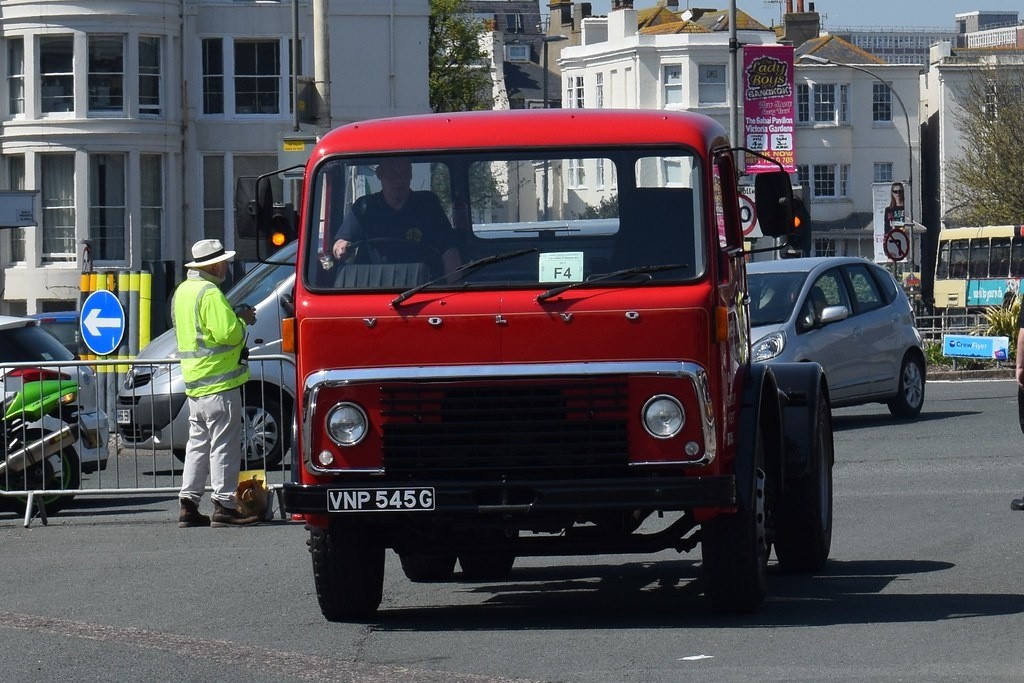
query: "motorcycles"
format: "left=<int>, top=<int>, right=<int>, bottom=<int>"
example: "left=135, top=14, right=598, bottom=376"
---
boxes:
left=0, top=366, right=80, bottom=521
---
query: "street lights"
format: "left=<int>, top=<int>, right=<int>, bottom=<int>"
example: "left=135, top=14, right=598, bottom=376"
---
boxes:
left=800, top=54, right=915, bottom=312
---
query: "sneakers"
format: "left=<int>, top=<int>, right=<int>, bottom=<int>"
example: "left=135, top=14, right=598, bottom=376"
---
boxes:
left=178, top=498, right=211, bottom=527
left=210, top=498, right=261, bottom=527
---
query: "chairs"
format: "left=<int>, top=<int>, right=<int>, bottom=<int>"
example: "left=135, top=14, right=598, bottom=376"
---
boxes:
left=812, top=287, right=827, bottom=315
left=614, top=185, right=695, bottom=275
left=416, top=190, right=454, bottom=235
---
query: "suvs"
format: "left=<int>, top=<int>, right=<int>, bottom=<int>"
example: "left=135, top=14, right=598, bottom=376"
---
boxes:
left=114, top=237, right=296, bottom=471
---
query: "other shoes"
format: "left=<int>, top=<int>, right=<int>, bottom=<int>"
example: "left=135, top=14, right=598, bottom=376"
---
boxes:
left=1011, top=497, right=1024, bottom=510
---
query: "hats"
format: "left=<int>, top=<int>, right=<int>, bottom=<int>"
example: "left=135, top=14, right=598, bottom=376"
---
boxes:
left=186, top=239, right=237, bottom=268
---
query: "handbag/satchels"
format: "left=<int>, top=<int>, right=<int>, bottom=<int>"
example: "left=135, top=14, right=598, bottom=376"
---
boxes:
left=237, top=475, right=275, bottom=520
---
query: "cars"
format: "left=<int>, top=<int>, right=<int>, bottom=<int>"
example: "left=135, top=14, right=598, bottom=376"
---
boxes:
left=745, top=257, right=928, bottom=423
left=0, top=310, right=110, bottom=475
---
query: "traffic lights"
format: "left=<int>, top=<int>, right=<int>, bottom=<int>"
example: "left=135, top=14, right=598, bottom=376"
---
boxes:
left=787, top=196, right=811, bottom=252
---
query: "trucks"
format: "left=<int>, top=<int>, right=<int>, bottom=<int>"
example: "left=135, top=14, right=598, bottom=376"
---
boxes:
left=254, top=106, right=839, bottom=621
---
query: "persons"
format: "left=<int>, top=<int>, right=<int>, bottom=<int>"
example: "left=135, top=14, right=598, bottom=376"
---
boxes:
left=884, top=183, right=904, bottom=234
left=170, top=239, right=256, bottom=528
left=1010, top=291, right=1024, bottom=510
left=788, top=281, right=826, bottom=316
left=332, top=162, right=462, bottom=286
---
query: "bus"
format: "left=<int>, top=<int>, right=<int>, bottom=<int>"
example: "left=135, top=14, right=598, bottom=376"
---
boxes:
left=933, top=225, right=1024, bottom=312
